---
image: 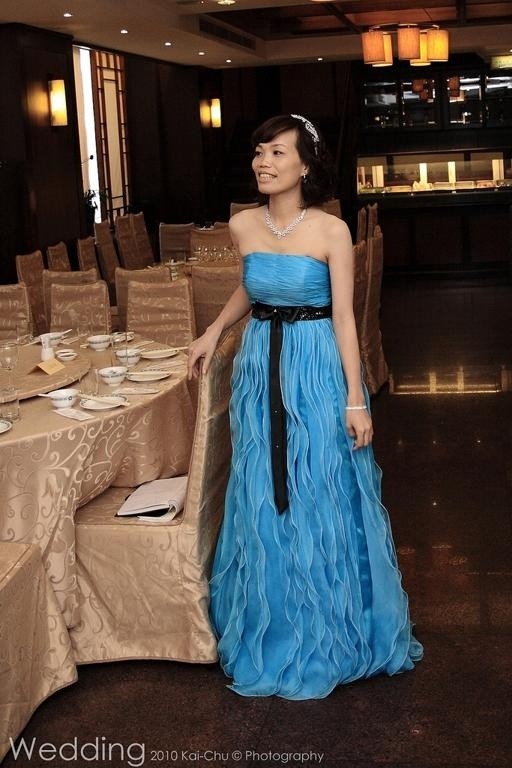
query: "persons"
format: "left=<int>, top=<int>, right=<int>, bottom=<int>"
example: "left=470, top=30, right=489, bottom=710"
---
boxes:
left=187, top=110, right=428, bottom=705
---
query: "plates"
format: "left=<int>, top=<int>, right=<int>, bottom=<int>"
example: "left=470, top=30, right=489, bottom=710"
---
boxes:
left=0, top=419, right=13, bottom=433
left=55, top=349, right=78, bottom=362
left=79, top=332, right=179, bottom=412
left=38, top=332, right=68, bottom=341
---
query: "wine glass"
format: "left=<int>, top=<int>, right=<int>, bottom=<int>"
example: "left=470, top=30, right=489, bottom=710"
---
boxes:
left=0, top=343, right=19, bottom=392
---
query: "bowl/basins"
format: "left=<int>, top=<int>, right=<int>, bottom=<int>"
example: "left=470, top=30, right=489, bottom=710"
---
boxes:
left=48, top=335, right=62, bottom=347
left=46, top=388, right=79, bottom=408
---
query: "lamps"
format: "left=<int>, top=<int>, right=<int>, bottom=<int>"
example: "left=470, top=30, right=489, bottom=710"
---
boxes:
left=362, top=23, right=450, bottom=68
left=372, top=166, right=377, bottom=187
left=210, top=98, right=222, bottom=128
left=448, top=161, right=456, bottom=183
left=500, top=159, right=504, bottom=180
left=419, top=162, right=427, bottom=184
left=492, top=159, right=499, bottom=180
left=48, top=79, right=68, bottom=127
left=411, top=76, right=460, bottom=100
left=377, top=165, right=384, bottom=187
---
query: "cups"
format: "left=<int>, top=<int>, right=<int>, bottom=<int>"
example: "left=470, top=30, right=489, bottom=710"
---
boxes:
left=0, top=388, right=20, bottom=422
left=41, top=348, right=54, bottom=361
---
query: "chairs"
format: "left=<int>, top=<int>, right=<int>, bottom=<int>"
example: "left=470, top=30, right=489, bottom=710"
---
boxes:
left=0, top=199, right=390, bottom=764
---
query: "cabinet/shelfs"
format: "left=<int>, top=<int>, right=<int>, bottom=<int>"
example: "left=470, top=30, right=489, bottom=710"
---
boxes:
left=360, top=64, right=512, bottom=134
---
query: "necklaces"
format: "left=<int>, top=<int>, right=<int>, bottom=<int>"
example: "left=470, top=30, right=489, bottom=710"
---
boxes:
left=263, top=206, right=308, bottom=240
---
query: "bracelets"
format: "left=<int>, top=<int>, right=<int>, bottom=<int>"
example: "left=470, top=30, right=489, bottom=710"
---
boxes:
left=344, top=405, right=371, bottom=411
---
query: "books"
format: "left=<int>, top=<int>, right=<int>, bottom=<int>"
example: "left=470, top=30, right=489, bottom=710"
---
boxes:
left=115, top=472, right=187, bottom=524
